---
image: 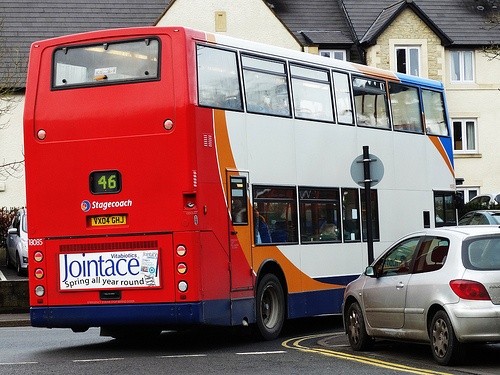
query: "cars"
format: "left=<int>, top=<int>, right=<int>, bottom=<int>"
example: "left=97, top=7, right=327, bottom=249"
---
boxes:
left=5, top=207, right=29, bottom=277
left=455, top=209, right=500, bottom=225
left=341, top=224, right=500, bottom=366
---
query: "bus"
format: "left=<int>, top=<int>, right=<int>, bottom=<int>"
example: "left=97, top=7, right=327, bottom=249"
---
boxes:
left=22, top=26, right=457, bottom=341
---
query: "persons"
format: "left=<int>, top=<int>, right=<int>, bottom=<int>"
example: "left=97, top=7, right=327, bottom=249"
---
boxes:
left=268, top=204, right=284, bottom=222
left=399, top=261, right=408, bottom=272
left=435, top=211, right=444, bottom=222
